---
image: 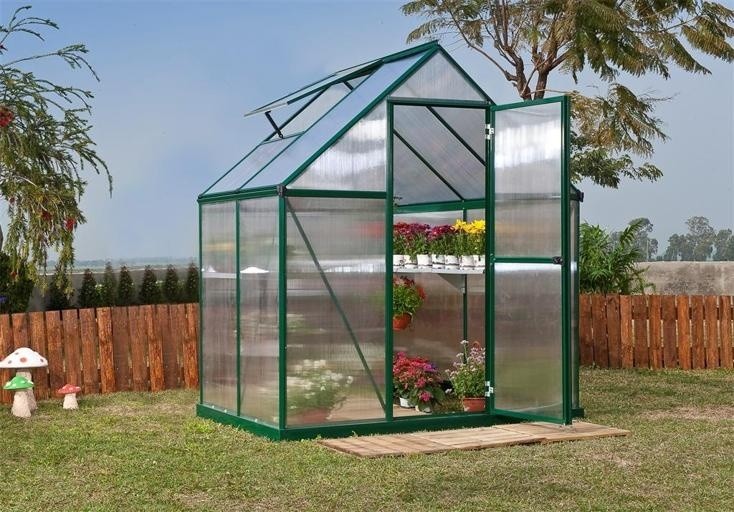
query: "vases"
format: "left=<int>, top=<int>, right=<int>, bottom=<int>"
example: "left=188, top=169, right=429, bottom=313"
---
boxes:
left=287, top=405, right=330, bottom=424
left=391, top=312, right=412, bottom=332
left=392, top=254, right=486, bottom=270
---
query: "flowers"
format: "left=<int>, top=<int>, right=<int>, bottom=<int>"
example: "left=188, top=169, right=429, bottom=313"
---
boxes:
left=392, top=277, right=426, bottom=324
left=274, top=355, right=354, bottom=414
left=390, top=338, right=485, bottom=414
left=388, top=218, right=488, bottom=264
